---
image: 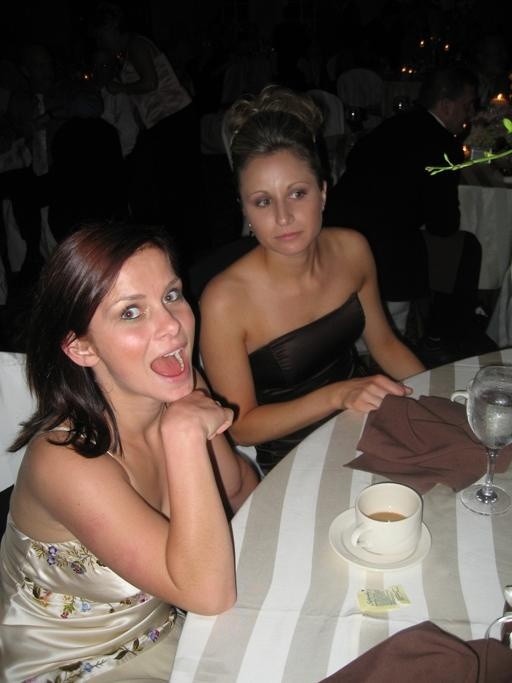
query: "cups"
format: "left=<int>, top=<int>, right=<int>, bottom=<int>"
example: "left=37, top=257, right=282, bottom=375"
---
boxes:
left=350, top=482, right=423, bottom=555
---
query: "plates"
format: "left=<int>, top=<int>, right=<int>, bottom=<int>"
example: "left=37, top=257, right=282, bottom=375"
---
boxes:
left=328, top=505, right=432, bottom=573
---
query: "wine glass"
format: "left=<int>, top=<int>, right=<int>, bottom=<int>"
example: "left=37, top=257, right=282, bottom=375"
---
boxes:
left=460, top=364, right=512, bottom=517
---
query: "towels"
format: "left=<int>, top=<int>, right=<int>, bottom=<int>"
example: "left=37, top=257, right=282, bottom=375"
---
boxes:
left=345, top=384, right=511, bottom=497
left=316, top=618, right=511, bottom=682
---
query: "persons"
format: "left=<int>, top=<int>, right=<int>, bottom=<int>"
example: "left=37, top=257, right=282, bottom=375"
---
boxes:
left=198, top=110, right=428, bottom=479
left=1, top=222, right=261, bottom=683
